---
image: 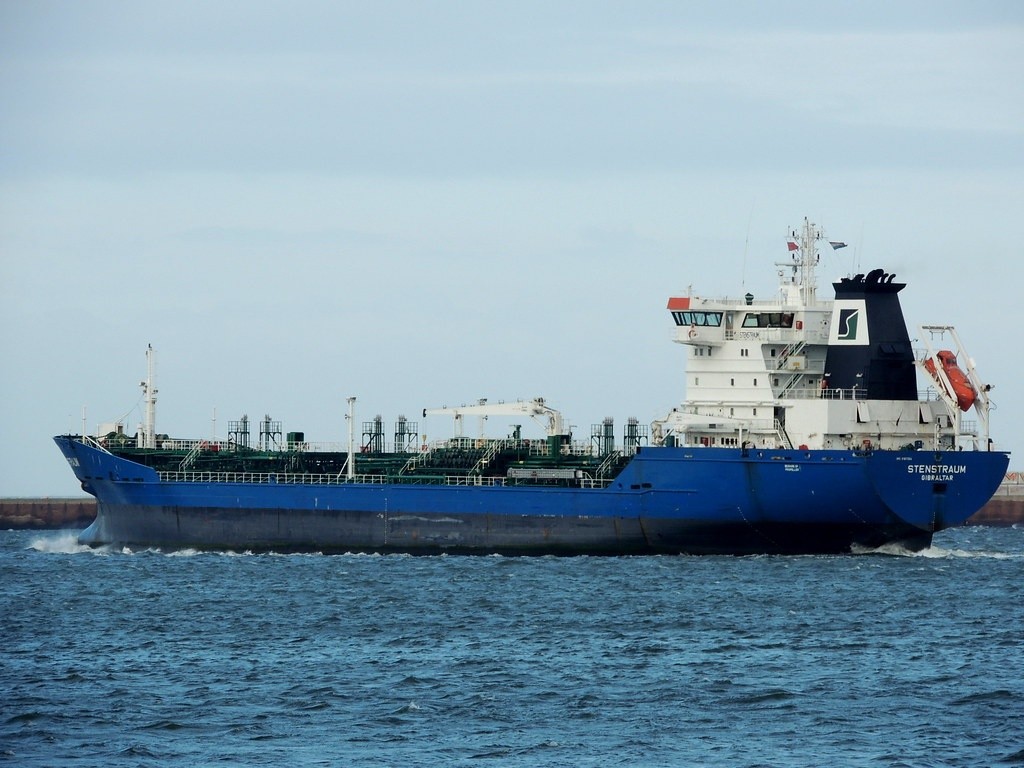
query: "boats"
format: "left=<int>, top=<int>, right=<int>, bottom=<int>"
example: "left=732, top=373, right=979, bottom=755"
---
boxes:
left=927, top=349, right=975, bottom=412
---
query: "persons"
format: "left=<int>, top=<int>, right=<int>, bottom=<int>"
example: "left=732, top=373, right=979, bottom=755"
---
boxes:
left=575, top=467, right=583, bottom=487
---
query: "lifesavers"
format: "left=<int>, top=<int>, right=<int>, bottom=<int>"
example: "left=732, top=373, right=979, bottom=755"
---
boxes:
left=688, top=327, right=697, bottom=338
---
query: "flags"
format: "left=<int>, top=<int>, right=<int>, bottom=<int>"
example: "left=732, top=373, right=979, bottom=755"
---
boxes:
left=788, top=241, right=799, bottom=252
left=830, top=241, right=848, bottom=251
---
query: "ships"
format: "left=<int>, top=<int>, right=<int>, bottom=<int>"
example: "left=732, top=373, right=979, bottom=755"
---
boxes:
left=49, top=212, right=1013, bottom=552
